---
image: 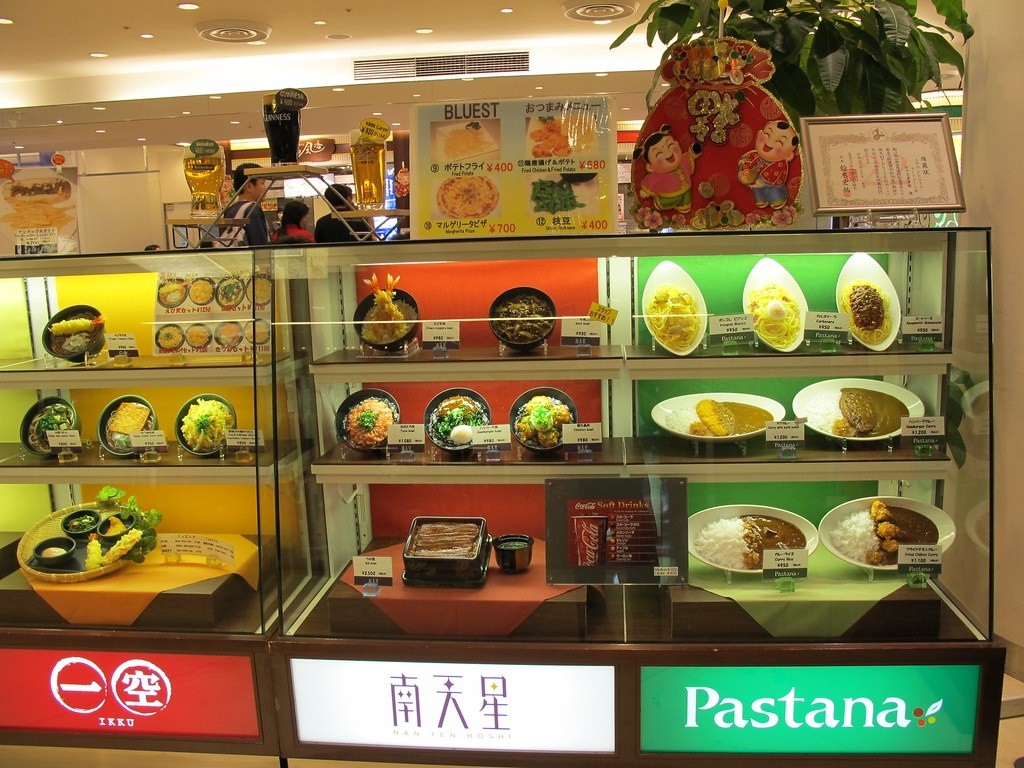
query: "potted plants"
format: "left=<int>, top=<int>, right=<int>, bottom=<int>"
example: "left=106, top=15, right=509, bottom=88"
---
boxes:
left=608, top=0, right=976, bottom=229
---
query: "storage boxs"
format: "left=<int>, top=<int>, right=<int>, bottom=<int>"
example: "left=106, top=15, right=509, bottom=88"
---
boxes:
left=402, top=516, right=489, bottom=584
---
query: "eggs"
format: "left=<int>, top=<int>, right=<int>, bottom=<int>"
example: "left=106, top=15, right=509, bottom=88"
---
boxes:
left=41, top=547, right=65, bottom=557
left=531, top=407, right=553, bottom=431
left=450, top=425, right=473, bottom=443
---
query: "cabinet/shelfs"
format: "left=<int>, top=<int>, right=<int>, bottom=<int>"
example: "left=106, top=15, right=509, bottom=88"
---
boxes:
left=308, top=344, right=968, bottom=492
left=0, top=353, right=306, bottom=488
left=164, top=165, right=409, bottom=249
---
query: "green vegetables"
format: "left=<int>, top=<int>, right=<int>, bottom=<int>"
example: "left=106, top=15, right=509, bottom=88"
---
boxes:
left=224, top=282, right=239, bottom=297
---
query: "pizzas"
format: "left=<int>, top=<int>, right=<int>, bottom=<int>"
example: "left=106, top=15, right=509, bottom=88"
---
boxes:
left=437, top=177, right=499, bottom=219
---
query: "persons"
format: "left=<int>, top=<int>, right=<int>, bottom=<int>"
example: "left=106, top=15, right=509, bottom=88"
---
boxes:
left=223, top=162, right=269, bottom=246
left=200, top=238, right=217, bottom=248
left=270, top=200, right=313, bottom=243
left=314, top=183, right=374, bottom=244
left=144, top=245, right=160, bottom=251
left=272, top=207, right=285, bottom=231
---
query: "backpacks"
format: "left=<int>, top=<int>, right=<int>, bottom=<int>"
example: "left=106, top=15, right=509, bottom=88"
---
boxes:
left=214, top=199, right=255, bottom=247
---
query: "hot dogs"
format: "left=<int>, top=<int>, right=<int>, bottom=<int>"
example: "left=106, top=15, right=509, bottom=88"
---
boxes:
left=1, top=177, right=72, bottom=203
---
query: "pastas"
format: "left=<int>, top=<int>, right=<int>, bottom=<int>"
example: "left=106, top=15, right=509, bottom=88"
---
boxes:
left=190, top=280, right=212, bottom=301
left=645, top=283, right=701, bottom=350
left=28, top=413, right=52, bottom=452
left=107, top=409, right=153, bottom=453
left=444, top=128, right=492, bottom=161
left=747, top=282, right=802, bottom=348
left=158, top=326, right=184, bottom=349
left=186, top=326, right=210, bottom=347
left=838, top=279, right=890, bottom=345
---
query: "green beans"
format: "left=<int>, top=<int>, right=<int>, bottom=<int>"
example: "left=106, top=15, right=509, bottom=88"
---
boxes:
left=531, top=177, right=586, bottom=214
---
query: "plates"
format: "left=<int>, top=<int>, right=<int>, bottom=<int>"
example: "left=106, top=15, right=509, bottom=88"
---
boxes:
left=835, top=252, right=901, bottom=352
left=791, top=377, right=925, bottom=441
left=0, top=169, right=78, bottom=253
left=688, top=503, right=819, bottom=574
left=641, top=260, right=708, bottom=356
left=436, top=120, right=500, bottom=163
left=817, top=495, right=957, bottom=571
left=650, top=392, right=787, bottom=442
left=742, top=257, right=809, bottom=352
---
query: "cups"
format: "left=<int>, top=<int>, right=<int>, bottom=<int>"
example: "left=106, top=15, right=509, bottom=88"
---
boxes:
left=182, top=145, right=227, bottom=219
left=350, top=129, right=387, bottom=211
left=571, top=515, right=608, bottom=567
left=260, top=92, right=301, bottom=167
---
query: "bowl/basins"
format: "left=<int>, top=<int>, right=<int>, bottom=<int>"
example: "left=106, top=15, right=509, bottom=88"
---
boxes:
left=97, top=394, right=157, bottom=457
left=154, top=274, right=272, bottom=351
left=19, top=395, right=81, bottom=456
left=423, top=386, right=492, bottom=454
left=335, top=388, right=401, bottom=454
left=33, top=536, right=76, bottom=567
left=173, top=392, right=237, bottom=456
left=42, top=304, right=105, bottom=363
left=353, top=289, right=419, bottom=352
left=96, top=512, right=137, bottom=543
left=509, top=387, right=579, bottom=454
left=488, top=285, right=556, bottom=350
left=492, top=534, right=534, bottom=574
left=61, top=508, right=101, bottom=539
left=402, top=515, right=487, bottom=584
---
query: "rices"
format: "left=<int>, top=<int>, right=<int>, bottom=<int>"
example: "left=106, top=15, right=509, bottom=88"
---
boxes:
left=694, top=517, right=751, bottom=570
left=801, top=390, right=856, bottom=436
left=664, top=404, right=714, bottom=437
left=829, top=507, right=880, bottom=563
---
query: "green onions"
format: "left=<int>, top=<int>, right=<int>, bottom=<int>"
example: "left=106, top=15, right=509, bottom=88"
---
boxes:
left=357, top=409, right=380, bottom=432
left=41, top=414, right=63, bottom=432
left=162, top=332, right=173, bottom=341
left=189, top=414, right=216, bottom=433
left=438, top=403, right=487, bottom=434
left=522, top=403, right=569, bottom=434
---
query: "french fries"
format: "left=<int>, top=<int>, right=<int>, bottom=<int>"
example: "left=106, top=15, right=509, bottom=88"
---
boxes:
left=0, top=202, right=76, bottom=231
